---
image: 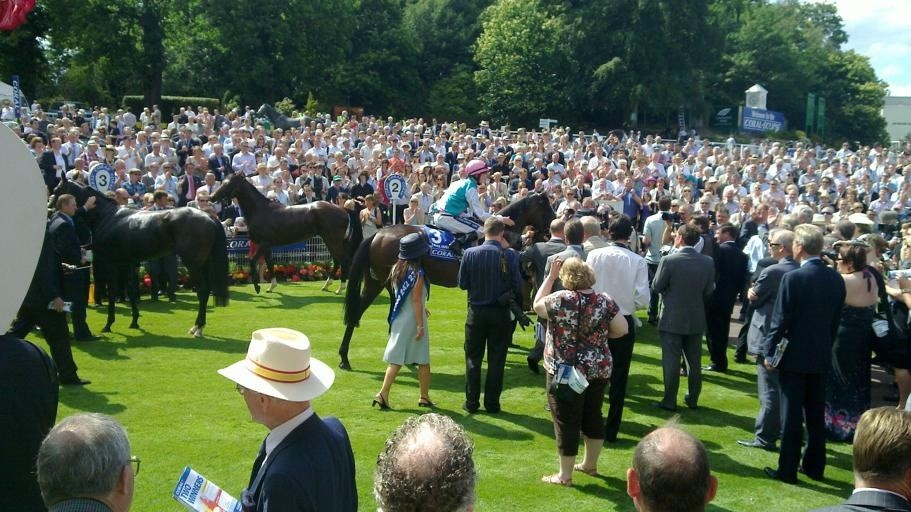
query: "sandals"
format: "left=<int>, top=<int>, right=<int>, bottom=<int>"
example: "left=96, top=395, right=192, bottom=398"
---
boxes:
left=542, top=472, right=573, bottom=486
left=574, top=461, right=597, bottom=475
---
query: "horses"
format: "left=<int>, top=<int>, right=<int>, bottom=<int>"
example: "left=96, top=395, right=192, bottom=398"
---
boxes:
left=338, top=190, right=559, bottom=372
left=255, top=103, right=318, bottom=136
left=47, top=168, right=230, bottom=341
left=207, top=165, right=365, bottom=296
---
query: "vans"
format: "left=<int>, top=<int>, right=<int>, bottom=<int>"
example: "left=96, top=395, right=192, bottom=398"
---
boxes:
left=0, top=81, right=30, bottom=116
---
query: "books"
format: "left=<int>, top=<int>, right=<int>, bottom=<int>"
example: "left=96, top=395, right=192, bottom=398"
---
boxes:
left=172, top=466, right=242, bottom=511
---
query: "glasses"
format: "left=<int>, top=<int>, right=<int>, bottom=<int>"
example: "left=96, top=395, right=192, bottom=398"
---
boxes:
left=236, top=384, right=249, bottom=396
left=128, top=455, right=141, bottom=476
left=768, top=240, right=781, bottom=248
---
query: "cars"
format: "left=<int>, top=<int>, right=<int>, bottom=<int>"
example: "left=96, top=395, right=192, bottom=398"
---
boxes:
left=713, top=107, right=734, bottom=129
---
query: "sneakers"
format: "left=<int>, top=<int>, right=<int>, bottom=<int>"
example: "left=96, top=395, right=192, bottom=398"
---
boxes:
left=527, top=356, right=540, bottom=372
left=463, top=400, right=476, bottom=413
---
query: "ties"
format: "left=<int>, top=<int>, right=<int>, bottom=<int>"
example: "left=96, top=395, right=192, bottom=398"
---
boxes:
left=72, top=144, right=76, bottom=165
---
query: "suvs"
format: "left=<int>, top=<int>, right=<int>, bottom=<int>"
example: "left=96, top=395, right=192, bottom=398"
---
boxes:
left=48, top=100, right=92, bottom=120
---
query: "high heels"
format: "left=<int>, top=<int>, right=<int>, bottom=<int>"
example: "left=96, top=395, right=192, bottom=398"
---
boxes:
left=418, top=395, right=437, bottom=408
left=371, top=392, right=391, bottom=409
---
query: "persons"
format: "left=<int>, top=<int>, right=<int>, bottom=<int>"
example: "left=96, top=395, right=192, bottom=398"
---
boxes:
left=1, top=334, right=142, bottom=512
left=2, top=97, right=911, bottom=486
left=218, top=327, right=358, bottom=512
left=815, top=406, right=910, bottom=510
left=373, top=412, right=479, bottom=511
left=628, top=413, right=719, bottom=511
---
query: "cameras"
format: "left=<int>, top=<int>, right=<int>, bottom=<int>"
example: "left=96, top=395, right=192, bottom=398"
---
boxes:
left=662, top=211, right=681, bottom=224
left=879, top=221, right=901, bottom=237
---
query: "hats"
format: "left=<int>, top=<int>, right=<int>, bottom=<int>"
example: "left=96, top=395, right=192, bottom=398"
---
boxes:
left=87, top=121, right=893, bottom=226
left=397, top=232, right=426, bottom=260
left=215, top=326, right=336, bottom=404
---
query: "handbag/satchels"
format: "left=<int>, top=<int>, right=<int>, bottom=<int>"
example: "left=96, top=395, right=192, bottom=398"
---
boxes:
left=548, top=359, right=591, bottom=408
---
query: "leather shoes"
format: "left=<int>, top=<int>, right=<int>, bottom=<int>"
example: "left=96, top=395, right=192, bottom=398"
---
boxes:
left=61, top=378, right=92, bottom=386
left=685, top=392, right=697, bottom=411
left=737, top=436, right=773, bottom=450
left=797, top=465, right=806, bottom=472
left=679, top=365, right=716, bottom=376
left=764, top=465, right=781, bottom=480
left=74, top=333, right=103, bottom=342
left=651, top=399, right=679, bottom=413
